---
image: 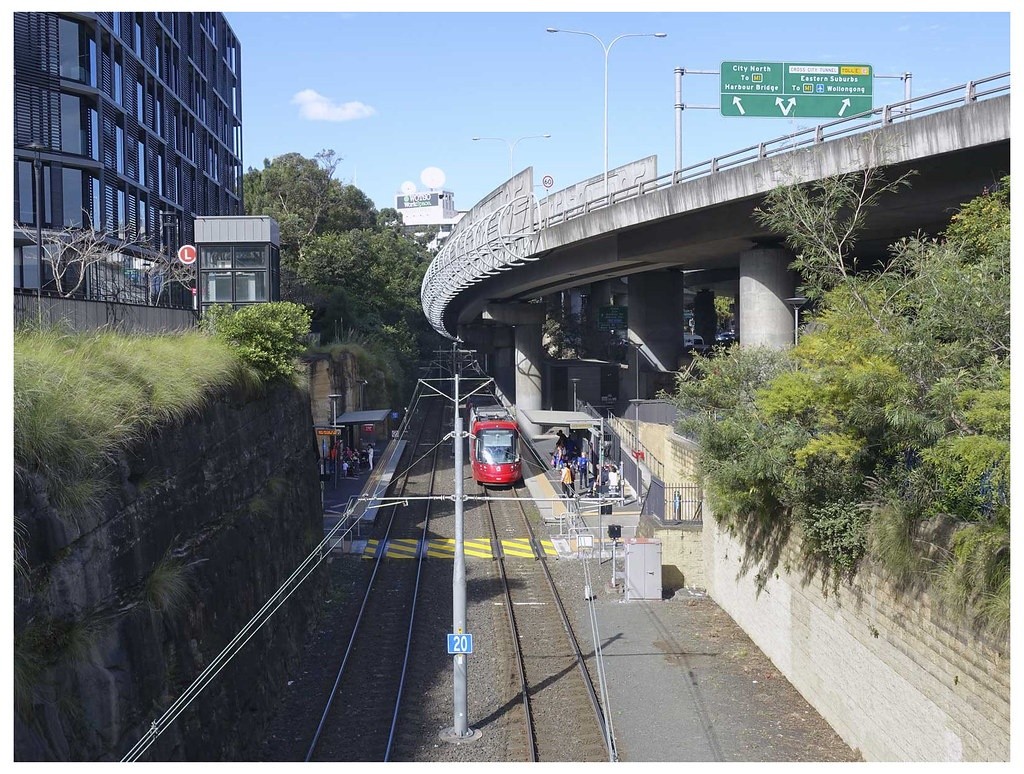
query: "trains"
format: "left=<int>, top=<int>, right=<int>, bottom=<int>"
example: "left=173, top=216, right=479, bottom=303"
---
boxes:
left=464, top=383, right=524, bottom=488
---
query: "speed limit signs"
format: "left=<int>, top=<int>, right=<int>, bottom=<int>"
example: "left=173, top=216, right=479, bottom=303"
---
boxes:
left=543, top=176, right=554, bottom=187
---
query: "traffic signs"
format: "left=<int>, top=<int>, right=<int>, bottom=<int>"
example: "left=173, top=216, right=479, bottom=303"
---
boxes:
left=718, top=60, right=874, bottom=120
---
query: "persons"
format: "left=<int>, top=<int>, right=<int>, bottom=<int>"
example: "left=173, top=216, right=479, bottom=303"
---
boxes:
left=331, top=443, right=374, bottom=477
left=550, top=430, right=609, bottom=498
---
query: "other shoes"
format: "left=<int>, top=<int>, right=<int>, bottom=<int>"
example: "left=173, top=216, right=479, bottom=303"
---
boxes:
left=587, top=491, right=592, bottom=496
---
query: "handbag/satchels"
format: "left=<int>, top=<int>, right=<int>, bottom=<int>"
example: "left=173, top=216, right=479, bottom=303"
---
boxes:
left=551, top=459, right=555, bottom=467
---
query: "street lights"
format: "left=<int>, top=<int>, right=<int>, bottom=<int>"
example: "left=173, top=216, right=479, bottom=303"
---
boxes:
left=356, top=379, right=367, bottom=413
left=547, top=26, right=668, bottom=205
left=327, top=392, right=343, bottom=444
left=471, top=134, right=552, bottom=178
